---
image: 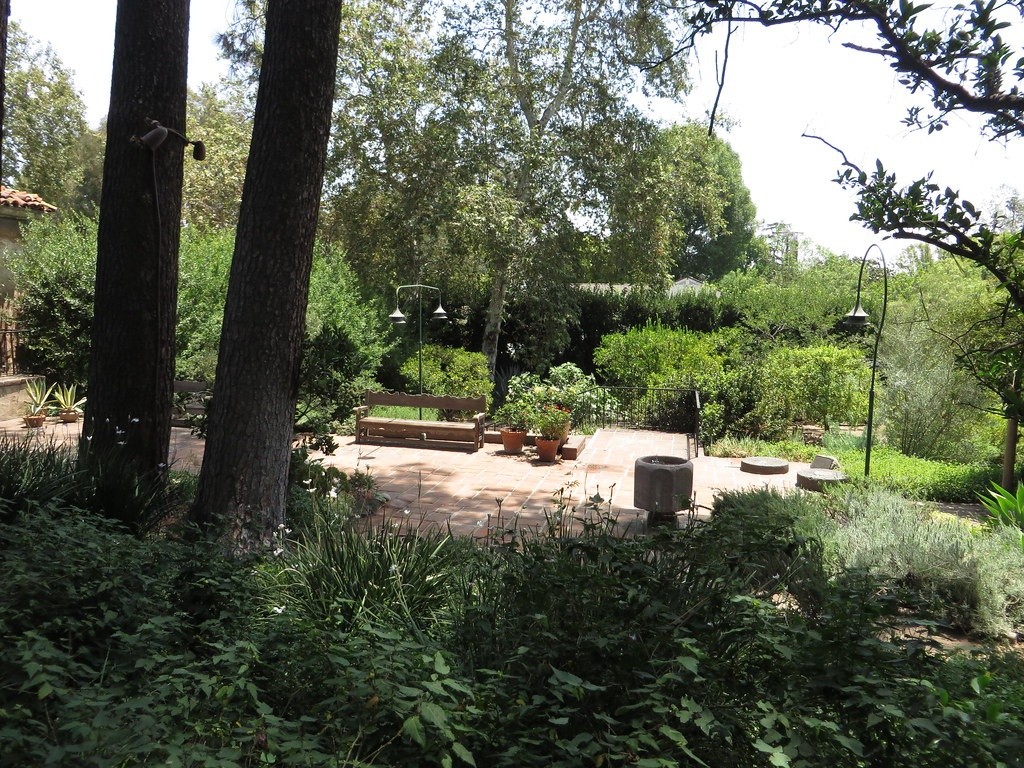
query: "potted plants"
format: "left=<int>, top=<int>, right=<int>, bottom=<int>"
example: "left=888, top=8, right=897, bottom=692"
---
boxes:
left=51, top=384, right=86, bottom=422
left=491, top=390, right=574, bottom=462
left=24, top=377, right=57, bottom=427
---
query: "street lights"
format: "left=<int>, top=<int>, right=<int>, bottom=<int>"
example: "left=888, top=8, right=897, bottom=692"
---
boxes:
left=131, top=117, right=229, bottom=483
left=844, top=244, right=887, bottom=475
left=388, top=284, right=447, bottom=420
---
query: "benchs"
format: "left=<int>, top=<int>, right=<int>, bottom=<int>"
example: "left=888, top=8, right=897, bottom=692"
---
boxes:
left=353, top=389, right=487, bottom=453
